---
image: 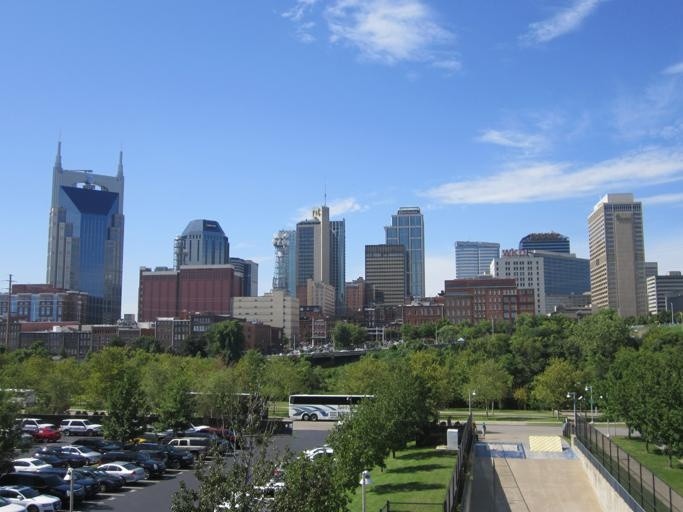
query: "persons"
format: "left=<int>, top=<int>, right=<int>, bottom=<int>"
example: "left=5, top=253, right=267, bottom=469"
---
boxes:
left=481, top=423, right=487, bottom=436
left=472, top=423, right=475, bottom=429
left=474, top=427, right=479, bottom=442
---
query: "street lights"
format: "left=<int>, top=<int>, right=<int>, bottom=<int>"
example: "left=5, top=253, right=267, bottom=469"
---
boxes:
left=467, top=389, right=475, bottom=414
left=599, top=394, right=609, bottom=438
left=357, top=469, right=373, bottom=512
left=577, top=395, right=583, bottom=415
left=566, top=391, right=576, bottom=435
left=584, top=384, right=593, bottom=424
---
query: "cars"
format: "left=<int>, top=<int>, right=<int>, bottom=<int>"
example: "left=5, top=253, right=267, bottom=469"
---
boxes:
left=273, top=340, right=394, bottom=357
left=0, top=411, right=243, bottom=512
left=214, top=443, right=334, bottom=512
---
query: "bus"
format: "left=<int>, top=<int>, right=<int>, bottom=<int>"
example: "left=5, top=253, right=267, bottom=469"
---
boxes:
left=157, top=387, right=268, bottom=426
left=0, top=386, right=38, bottom=412
left=287, top=394, right=374, bottom=425
